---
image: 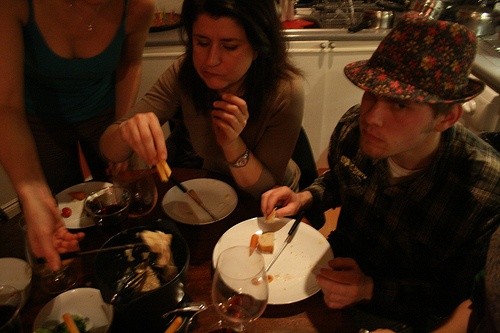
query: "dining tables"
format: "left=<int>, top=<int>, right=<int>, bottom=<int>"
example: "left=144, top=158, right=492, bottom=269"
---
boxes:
left=0, top=167, right=362, bottom=333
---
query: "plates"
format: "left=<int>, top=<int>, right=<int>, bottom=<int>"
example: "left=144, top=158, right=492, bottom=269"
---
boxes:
left=212, top=217, right=334, bottom=305
left=56, top=181, right=114, bottom=229
left=0, top=256, right=33, bottom=294
left=161, top=178, right=238, bottom=225
left=31, top=288, right=112, bottom=333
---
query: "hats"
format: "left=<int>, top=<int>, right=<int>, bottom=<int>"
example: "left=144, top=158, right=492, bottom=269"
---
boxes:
left=344, top=12, right=486, bottom=102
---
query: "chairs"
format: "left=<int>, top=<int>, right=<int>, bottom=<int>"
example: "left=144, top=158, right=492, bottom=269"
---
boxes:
left=291, top=126, right=327, bottom=231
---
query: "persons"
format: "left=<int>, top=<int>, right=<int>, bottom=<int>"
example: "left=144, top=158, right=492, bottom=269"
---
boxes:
left=0, top=0, right=154, bottom=271
left=261, top=11, right=500, bottom=333
left=99, top=1, right=304, bottom=198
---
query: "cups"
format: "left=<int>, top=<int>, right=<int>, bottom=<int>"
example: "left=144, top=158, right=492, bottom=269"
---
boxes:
left=0, top=283, right=28, bottom=333
left=85, top=186, right=128, bottom=231
left=115, top=172, right=157, bottom=218
left=38, top=259, right=82, bottom=295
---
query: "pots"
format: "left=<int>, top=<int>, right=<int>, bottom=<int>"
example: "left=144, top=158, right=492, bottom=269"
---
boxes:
left=94, top=227, right=192, bottom=316
left=348, top=6, right=394, bottom=35
left=451, top=4, right=497, bottom=35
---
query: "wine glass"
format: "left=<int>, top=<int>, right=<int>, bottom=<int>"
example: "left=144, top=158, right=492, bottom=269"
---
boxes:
left=213, top=245, right=269, bottom=333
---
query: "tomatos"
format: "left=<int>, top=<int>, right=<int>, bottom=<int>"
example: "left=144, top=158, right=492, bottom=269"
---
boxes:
left=61, top=207, right=72, bottom=218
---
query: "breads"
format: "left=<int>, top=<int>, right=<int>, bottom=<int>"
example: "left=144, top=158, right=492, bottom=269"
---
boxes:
left=249, top=231, right=275, bottom=256
left=265, top=207, right=277, bottom=224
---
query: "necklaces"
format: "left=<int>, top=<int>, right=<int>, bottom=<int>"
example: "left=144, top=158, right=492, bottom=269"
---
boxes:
left=66, top=3, right=96, bottom=31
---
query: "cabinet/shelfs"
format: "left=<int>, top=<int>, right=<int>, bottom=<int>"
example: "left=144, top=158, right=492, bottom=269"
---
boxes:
left=285, top=40, right=382, bottom=163
left=456, top=74, right=500, bottom=138
left=134, top=45, right=186, bottom=141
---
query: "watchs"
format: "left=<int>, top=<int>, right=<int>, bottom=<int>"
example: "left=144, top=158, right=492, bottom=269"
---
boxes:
left=225, top=149, right=249, bottom=167
